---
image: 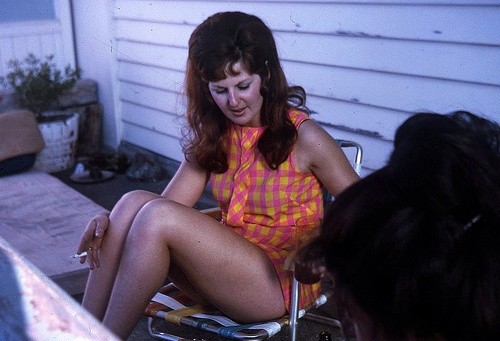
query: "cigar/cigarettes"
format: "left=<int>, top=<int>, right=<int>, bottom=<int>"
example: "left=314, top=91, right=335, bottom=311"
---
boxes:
left=72, top=251, right=87, bottom=258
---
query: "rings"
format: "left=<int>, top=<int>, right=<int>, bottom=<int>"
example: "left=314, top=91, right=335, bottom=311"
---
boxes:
left=87, top=247, right=92, bottom=250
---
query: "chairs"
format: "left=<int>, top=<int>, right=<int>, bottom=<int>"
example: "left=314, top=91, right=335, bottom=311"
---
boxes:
left=141, top=139, right=363, bottom=341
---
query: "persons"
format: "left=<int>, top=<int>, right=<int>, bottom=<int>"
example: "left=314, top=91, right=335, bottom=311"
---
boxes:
left=76, top=11, right=363, bottom=340
left=295, top=110, right=499, bottom=340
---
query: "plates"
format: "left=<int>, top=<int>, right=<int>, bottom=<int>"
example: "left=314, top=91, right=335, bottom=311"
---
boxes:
left=69, top=171, right=113, bottom=182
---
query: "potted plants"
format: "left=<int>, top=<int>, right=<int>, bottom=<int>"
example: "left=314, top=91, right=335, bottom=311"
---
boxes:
left=0, top=51, right=84, bottom=173
